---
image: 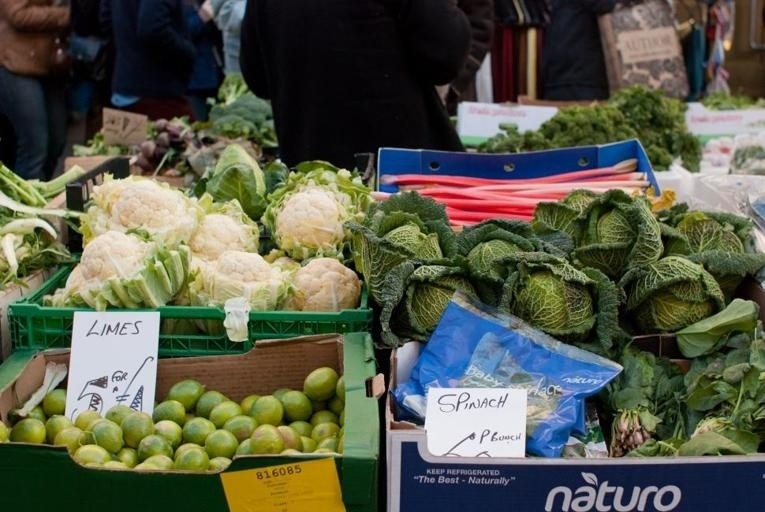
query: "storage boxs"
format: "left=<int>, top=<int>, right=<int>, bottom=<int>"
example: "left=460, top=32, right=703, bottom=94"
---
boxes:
left=0, top=328, right=386, bottom=511
left=384, top=340, right=765, bottom=511
left=43, top=172, right=104, bottom=246
left=0, top=265, right=59, bottom=362
left=376, top=137, right=661, bottom=197
left=64, top=137, right=263, bottom=187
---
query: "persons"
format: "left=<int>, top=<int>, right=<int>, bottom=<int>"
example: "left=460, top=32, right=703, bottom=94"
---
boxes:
left=0, top=0, right=622, bottom=180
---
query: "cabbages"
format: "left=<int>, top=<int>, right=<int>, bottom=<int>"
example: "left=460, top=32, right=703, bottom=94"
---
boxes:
left=340, top=188, right=765, bottom=357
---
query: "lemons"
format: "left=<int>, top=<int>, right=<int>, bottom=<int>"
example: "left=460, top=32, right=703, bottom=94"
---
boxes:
left=2, top=367, right=350, bottom=474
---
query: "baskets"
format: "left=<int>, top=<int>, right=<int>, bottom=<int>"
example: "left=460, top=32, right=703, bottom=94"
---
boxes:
left=4, top=250, right=372, bottom=351
left=375, top=137, right=660, bottom=230
left=65, top=156, right=131, bottom=249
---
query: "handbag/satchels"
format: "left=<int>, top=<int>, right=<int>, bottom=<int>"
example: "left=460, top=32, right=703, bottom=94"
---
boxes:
left=598, top=2, right=690, bottom=105
left=50, top=26, right=106, bottom=84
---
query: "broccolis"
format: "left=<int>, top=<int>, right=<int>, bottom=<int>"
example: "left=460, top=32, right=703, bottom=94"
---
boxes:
left=54, top=161, right=363, bottom=311
